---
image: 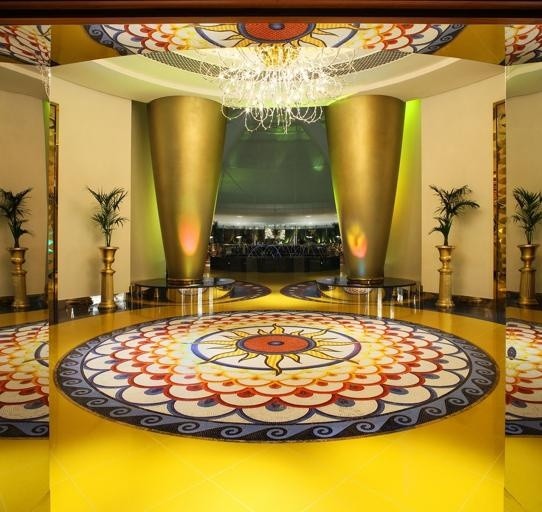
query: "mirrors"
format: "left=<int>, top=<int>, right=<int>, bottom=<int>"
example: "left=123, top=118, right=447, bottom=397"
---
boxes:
left=1, top=24, right=50, bottom=512
left=505, top=26, right=542, bottom=508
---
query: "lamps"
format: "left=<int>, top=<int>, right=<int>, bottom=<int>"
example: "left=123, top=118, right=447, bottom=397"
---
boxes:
left=198, top=46, right=356, bottom=135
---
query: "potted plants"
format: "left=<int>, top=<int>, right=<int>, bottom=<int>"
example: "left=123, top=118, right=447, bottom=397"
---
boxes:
left=88, top=188, right=127, bottom=311
left=427, top=182, right=481, bottom=312
left=0, top=184, right=35, bottom=314
left=512, top=186, right=542, bottom=306
